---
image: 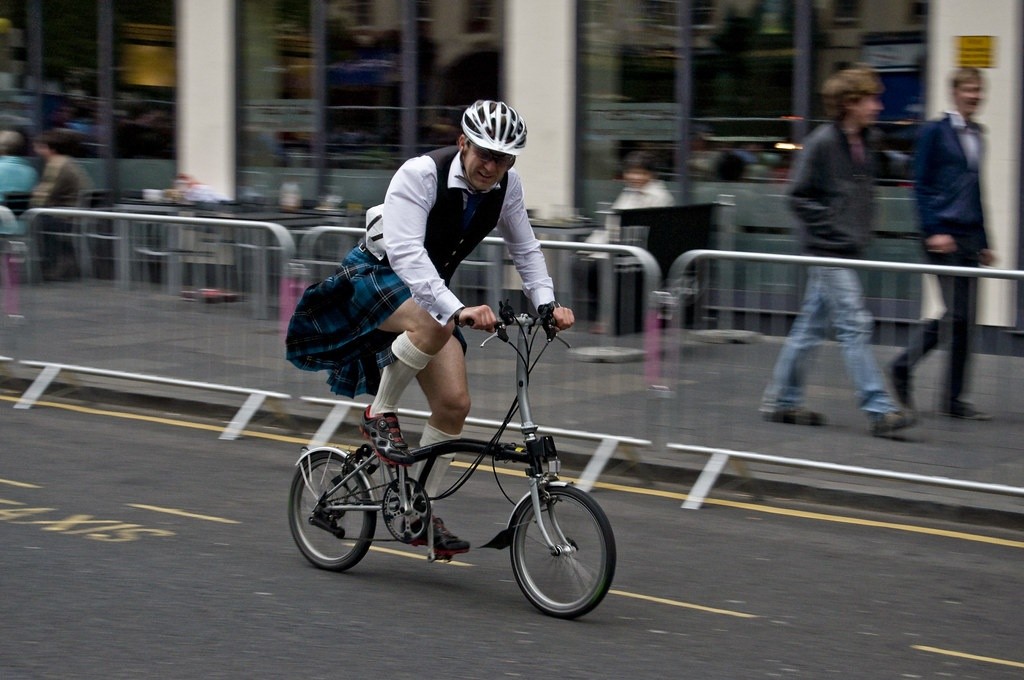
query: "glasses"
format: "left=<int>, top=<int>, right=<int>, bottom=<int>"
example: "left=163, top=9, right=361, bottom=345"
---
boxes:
left=465, top=137, right=513, bottom=167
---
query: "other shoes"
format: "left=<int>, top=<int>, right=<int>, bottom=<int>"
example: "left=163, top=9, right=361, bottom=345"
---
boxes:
left=887, top=365, right=908, bottom=405
left=869, top=412, right=917, bottom=434
left=763, top=408, right=821, bottom=426
left=936, top=404, right=991, bottom=420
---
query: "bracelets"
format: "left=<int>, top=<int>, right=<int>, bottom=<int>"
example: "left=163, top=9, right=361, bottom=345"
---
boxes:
left=453, top=307, right=466, bottom=328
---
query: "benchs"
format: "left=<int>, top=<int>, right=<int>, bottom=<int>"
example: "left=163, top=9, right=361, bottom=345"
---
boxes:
left=587, top=195, right=735, bottom=337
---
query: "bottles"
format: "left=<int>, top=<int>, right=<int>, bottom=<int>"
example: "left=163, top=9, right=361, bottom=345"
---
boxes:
left=175, top=174, right=188, bottom=189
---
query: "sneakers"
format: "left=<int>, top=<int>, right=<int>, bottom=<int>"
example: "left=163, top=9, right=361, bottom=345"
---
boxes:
left=359, top=405, right=416, bottom=466
left=412, top=515, right=470, bottom=555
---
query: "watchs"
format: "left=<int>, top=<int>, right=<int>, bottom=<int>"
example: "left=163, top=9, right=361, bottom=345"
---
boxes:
left=548, top=301, right=561, bottom=308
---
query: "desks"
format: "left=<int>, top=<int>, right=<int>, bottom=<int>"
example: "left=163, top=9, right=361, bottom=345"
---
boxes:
left=113, top=200, right=604, bottom=319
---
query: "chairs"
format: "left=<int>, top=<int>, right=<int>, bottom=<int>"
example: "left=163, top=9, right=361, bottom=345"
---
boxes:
left=0, top=189, right=215, bottom=303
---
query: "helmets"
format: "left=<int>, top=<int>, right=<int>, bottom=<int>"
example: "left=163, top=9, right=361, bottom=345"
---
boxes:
left=461, top=100, right=527, bottom=156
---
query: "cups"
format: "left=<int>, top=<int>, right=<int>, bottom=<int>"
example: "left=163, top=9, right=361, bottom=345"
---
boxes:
left=279, top=184, right=300, bottom=207
left=142, top=189, right=163, bottom=199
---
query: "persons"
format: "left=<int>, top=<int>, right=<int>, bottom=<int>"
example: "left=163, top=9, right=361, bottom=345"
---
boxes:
left=883, top=66, right=996, bottom=421
left=573, top=152, right=678, bottom=335
left=685, top=132, right=914, bottom=189
left=757, top=69, right=920, bottom=436
left=285, top=102, right=575, bottom=555
left=49, top=78, right=177, bottom=157
left=0, top=131, right=95, bottom=280
left=342, top=14, right=501, bottom=151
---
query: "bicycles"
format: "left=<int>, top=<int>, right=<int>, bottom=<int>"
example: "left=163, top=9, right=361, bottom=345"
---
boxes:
left=287, top=298, right=619, bottom=620
